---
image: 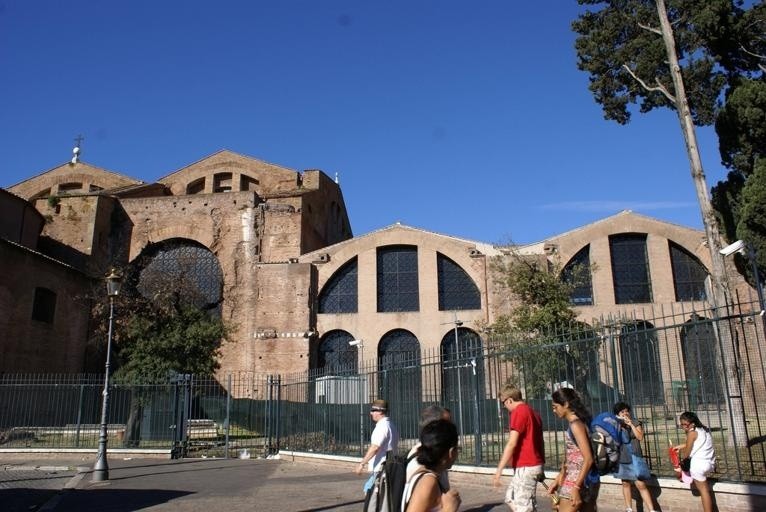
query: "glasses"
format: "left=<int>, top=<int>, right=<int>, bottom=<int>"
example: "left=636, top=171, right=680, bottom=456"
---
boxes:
left=455, top=446, right=462, bottom=454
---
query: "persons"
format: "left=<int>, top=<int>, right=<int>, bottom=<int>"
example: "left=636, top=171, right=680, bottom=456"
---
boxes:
left=547, top=386, right=601, bottom=512
left=404, top=403, right=452, bottom=494
left=612, top=402, right=658, bottom=512
left=491, top=383, right=546, bottom=512
left=398, top=418, right=463, bottom=512
left=672, top=411, right=720, bottom=512
left=357, top=398, right=400, bottom=496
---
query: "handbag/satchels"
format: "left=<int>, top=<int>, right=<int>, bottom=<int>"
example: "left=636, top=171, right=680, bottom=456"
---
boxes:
left=680, top=457, right=691, bottom=472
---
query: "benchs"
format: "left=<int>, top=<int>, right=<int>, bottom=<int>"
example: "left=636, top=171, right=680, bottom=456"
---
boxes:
left=187, top=419, right=218, bottom=438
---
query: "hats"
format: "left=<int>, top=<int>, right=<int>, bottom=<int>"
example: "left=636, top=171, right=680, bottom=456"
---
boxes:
left=370, top=399, right=390, bottom=411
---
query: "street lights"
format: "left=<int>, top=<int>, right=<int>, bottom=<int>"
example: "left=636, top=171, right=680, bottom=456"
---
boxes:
left=91, top=269, right=125, bottom=480
left=455, top=321, right=462, bottom=446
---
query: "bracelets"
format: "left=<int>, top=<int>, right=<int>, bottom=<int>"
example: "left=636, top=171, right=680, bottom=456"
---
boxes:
left=575, top=483, right=582, bottom=490
left=360, top=462, right=365, bottom=466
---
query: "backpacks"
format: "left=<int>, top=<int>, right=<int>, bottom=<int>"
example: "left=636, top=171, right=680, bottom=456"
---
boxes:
left=363, top=448, right=418, bottom=512
left=568, top=412, right=623, bottom=477
